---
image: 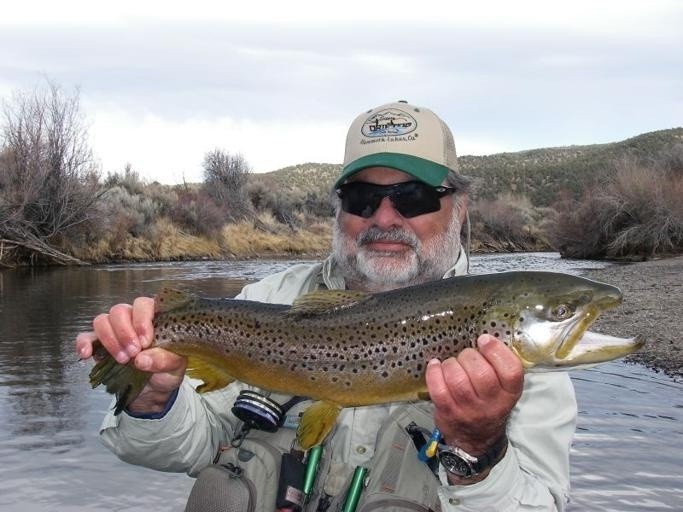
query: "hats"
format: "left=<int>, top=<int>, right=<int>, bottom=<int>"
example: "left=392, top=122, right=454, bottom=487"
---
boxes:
left=333, top=99, right=460, bottom=188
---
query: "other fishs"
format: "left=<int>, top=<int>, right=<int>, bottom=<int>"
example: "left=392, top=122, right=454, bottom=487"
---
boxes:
left=76, top=271, right=646, bottom=450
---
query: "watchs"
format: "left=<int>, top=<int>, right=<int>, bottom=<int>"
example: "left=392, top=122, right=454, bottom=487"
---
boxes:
left=434, top=434, right=508, bottom=478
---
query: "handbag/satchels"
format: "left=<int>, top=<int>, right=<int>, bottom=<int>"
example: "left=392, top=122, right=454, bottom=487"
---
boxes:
left=184, top=419, right=312, bottom=512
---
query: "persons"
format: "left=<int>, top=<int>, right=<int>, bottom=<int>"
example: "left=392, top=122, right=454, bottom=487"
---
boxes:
left=73, top=101, right=579, bottom=511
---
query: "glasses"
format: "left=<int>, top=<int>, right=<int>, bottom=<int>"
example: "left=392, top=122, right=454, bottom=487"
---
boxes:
left=335, top=179, right=457, bottom=220
